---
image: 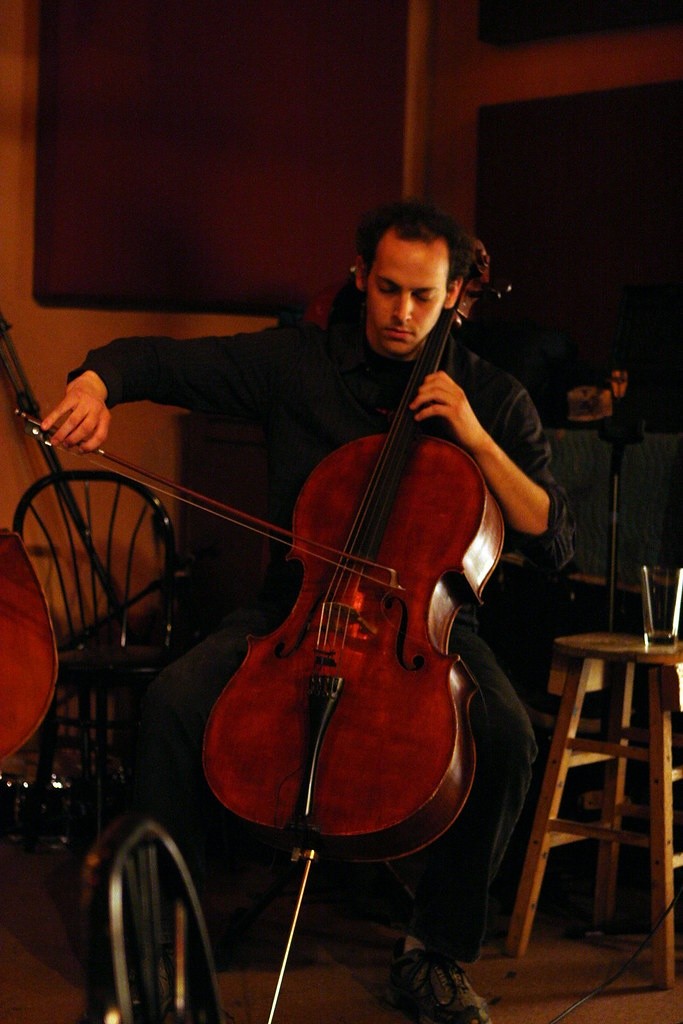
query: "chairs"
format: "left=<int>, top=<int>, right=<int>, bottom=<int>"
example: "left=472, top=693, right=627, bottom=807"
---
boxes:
left=13, top=470, right=192, bottom=855
left=80, top=813, right=226, bottom=1024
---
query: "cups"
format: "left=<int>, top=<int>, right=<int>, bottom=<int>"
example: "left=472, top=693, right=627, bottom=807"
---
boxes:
left=639, top=564, right=682, bottom=655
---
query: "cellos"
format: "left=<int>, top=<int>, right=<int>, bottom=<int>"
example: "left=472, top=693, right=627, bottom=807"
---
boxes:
left=1, top=524, right=62, bottom=762
left=198, top=235, right=512, bottom=1024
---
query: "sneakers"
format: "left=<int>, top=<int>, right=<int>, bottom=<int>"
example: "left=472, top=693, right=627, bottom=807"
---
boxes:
left=127, top=948, right=176, bottom=1024
left=379, top=937, right=492, bottom=1024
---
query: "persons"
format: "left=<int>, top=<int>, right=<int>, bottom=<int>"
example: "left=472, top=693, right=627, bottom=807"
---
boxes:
left=41, top=204, right=574, bottom=1024
left=326, top=234, right=641, bottom=930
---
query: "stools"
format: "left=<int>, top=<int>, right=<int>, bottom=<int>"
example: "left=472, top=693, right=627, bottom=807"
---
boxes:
left=502, top=630, right=683, bottom=991
